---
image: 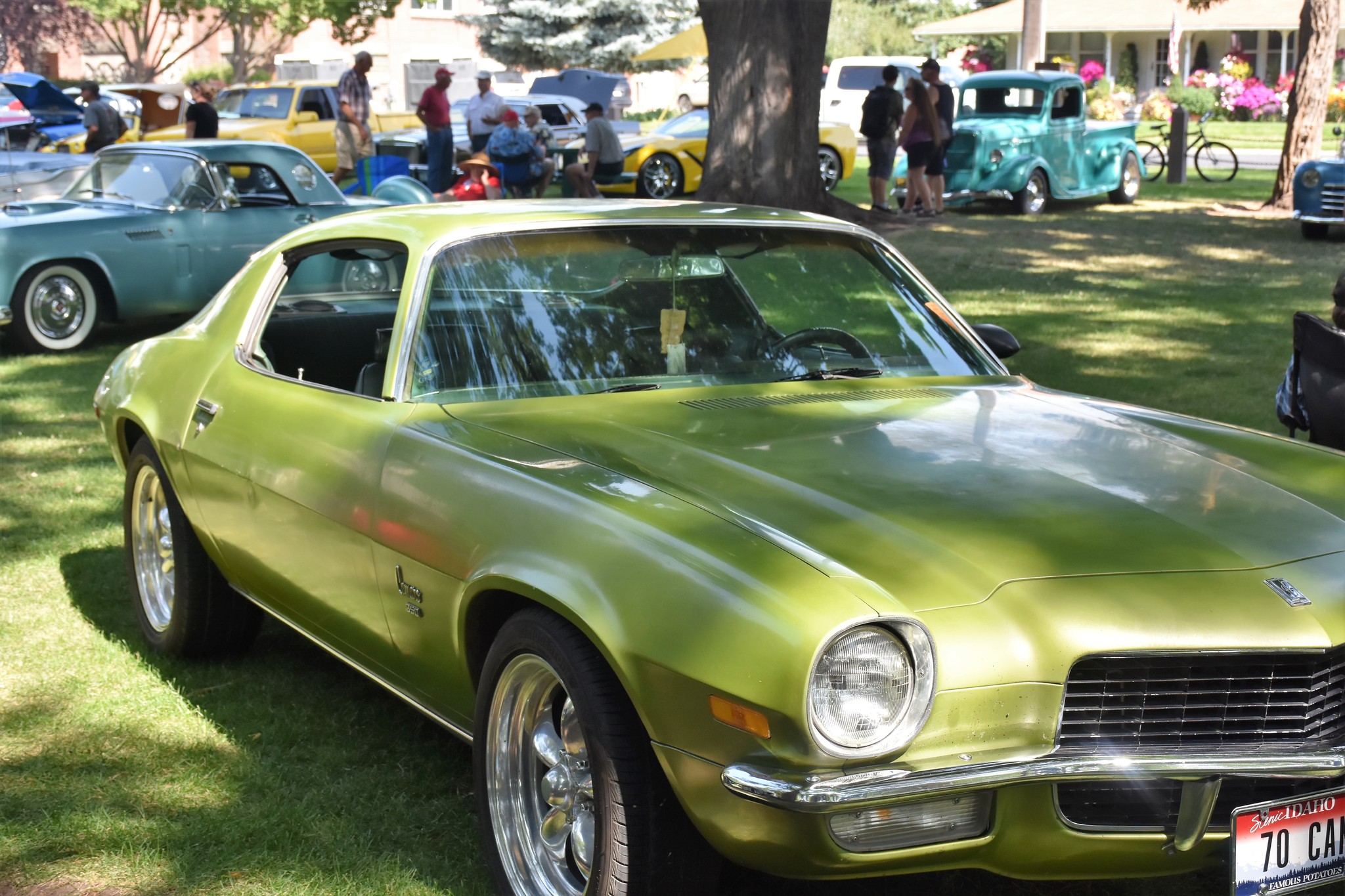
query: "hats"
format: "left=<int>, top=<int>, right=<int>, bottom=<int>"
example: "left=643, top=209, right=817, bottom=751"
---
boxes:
left=435, top=67, right=453, bottom=80
left=521, top=105, right=542, bottom=116
left=502, top=110, right=518, bottom=122
left=917, top=59, right=940, bottom=71
left=458, top=152, right=498, bottom=176
left=476, top=71, right=493, bottom=79
left=581, top=102, right=603, bottom=112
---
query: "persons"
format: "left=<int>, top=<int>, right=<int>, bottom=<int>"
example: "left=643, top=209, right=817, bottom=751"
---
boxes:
left=415, top=69, right=455, bottom=194
left=184, top=80, right=219, bottom=143
left=486, top=102, right=555, bottom=199
left=434, top=152, right=502, bottom=204
left=328, top=51, right=377, bottom=187
left=80, top=81, right=127, bottom=160
left=564, top=102, right=624, bottom=198
left=859, top=65, right=905, bottom=213
left=914, top=59, right=954, bottom=216
left=467, top=70, right=505, bottom=155
left=895, top=77, right=940, bottom=216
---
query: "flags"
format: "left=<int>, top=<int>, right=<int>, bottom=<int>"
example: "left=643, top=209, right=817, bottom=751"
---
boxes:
left=1165, top=15, right=1185, bottom=74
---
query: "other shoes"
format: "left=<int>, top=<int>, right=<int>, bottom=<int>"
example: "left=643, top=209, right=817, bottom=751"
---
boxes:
left=870, top=203, right=898, bottom=216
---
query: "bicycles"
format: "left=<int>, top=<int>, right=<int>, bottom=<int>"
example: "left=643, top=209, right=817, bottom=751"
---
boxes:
left=1131, top=109, right=1239, bottom=182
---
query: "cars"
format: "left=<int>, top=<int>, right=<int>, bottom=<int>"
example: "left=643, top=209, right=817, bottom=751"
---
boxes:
left=0, top=137, right=440, bottom=354
left=0, top=70, right=193, bottom=156
left=368, top=93, right=642, bottom=168
left=1292, top=126, right=1345, bottom=239
left=681, top=71, right=711, bottom=108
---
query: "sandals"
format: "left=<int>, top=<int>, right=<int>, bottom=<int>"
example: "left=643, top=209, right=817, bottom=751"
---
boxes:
left=916, top=209, right=935, bottom=217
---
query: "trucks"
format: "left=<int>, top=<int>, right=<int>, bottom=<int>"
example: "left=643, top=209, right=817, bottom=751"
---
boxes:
left=816, top=56, right=973, bottom=144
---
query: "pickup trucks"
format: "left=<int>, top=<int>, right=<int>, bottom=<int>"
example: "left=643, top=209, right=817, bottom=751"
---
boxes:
left=890, top=68, right=1147, bottom=215
left=140, top=79, right=426, bottom=194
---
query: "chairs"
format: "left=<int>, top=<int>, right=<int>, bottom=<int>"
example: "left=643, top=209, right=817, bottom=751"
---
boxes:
left=356, top=325, right=490, bottom=397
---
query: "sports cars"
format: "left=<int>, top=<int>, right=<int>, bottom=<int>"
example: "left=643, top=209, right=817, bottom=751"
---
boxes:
left=555, top=109, right=860, bottom=200
left=87, top=197, right=1345, bottom=896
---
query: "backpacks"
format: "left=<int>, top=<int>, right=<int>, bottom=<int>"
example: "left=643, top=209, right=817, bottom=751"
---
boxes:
left=860, top=88, right=894, bottom=140
